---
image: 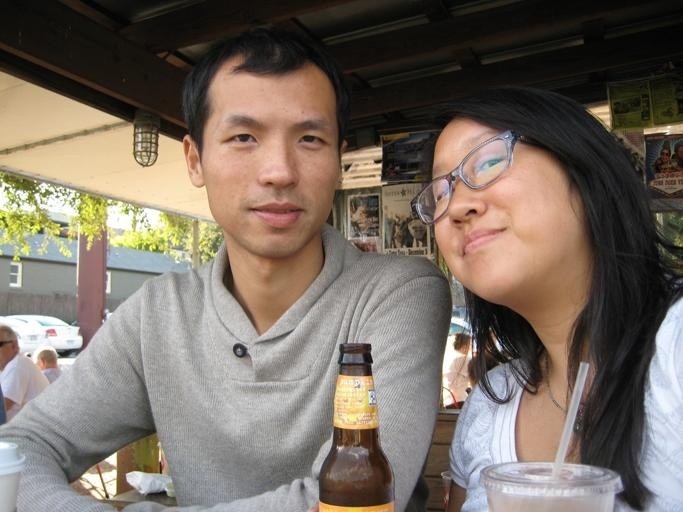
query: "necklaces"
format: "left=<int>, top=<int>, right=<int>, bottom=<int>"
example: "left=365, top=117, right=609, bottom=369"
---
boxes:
left=542, top=352, right=587, bottom=436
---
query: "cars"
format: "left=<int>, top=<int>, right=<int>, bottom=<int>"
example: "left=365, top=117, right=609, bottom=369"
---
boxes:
left=0, top=314, right=83, bottom=358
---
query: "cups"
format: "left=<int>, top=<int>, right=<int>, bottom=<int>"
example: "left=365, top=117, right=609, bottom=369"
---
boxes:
left=477, top=460, right=625, bottom=512
left=0, top=440, right=26, bottom=512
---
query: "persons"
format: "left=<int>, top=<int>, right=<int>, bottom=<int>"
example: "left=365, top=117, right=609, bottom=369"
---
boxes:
left=670, top=140, right=683, bottom=170
left=0, top=323, right=56, bottom=426
left=0, top=23, right=454, bottom=511
left=37, top=348, right=64, bottom=384
left=464, top=351, right=502, bottom=391
left=652, top=143, right=683, bottom=172
left=434, top=331, right=479, bottom=407
left=422, top=79, right=683, bottom=512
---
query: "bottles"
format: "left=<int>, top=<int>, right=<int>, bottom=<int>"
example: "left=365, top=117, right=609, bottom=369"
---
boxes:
left=316, top=339, right=396, bottom=512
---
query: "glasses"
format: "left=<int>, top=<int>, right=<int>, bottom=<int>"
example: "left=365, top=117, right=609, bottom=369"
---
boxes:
left=0, top=340, right=12, bottom=347
left=410, top=128, right=555, bottom=227
